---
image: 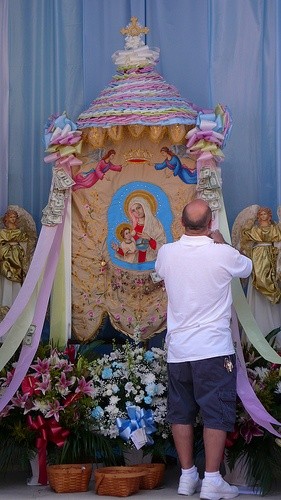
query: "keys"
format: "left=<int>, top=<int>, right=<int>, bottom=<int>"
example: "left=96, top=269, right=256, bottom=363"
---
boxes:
left=223, top=358, right=233, bottom=373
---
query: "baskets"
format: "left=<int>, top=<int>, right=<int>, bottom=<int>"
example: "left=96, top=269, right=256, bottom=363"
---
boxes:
left=94, top=467, right=146, bottom=497
left=46, top=464, right=90, bottom=492
left=133, top=463, right=165, bottom=489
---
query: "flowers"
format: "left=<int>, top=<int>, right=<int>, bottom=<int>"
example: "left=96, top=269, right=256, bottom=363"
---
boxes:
left=0, top=323, right=281, bottom=451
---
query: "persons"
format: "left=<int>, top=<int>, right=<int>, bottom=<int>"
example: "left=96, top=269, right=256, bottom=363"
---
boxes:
left=241, top=208, right=281, bottom=304
left=154, top=200, right=252, bottom=500
left=0, top=210, right=29, bottom=282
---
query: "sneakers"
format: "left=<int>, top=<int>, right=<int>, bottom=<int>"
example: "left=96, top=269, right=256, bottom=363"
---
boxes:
left=200, top=477, right=240, bottom=500
left=177, top=473, right=203, bottom=494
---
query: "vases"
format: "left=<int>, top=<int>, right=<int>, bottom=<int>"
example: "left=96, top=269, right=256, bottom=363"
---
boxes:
left=27, top=448, right=51, bottom=485
left=223, top=448, right=264, bottom=487
left=122, top=447, right=154, bottom=467
